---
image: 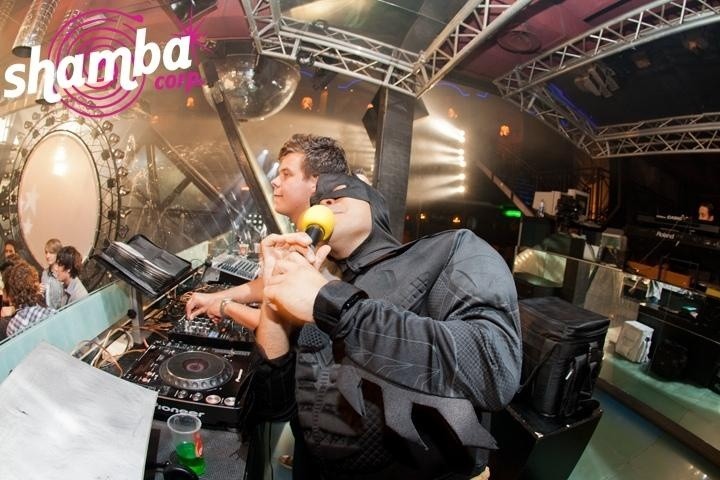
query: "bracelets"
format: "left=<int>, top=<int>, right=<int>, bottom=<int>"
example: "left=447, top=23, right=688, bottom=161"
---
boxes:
left=220, top=298, right=236, bottom=318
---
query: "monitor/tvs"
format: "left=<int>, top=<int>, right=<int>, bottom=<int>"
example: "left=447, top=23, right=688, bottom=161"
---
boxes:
left=532, top=191, right=573, bottom=217
left=568, top=188, right=590, bottom=221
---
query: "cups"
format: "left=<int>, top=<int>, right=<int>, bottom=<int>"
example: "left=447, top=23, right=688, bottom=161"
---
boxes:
left=167, top=413, right=206, bottom=475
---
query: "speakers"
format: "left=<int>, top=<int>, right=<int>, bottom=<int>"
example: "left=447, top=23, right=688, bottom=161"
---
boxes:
left=487, top=399, right=604, bottom=480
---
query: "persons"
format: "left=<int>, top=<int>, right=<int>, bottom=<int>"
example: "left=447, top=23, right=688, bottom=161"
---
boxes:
left=233, top=175, right=523, bottom=480
left=698, top=203, right=715, bottom=222
left=185, top=133, right=352, bottom=333
left=0, top=239, right=88, bottom=343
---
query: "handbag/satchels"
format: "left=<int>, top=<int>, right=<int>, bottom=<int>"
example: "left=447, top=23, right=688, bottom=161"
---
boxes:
left=511, top=295, right=610, bottom=416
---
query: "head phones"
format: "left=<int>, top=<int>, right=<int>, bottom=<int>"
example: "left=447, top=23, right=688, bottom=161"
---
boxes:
left=156, top=462, right=200, bottom=480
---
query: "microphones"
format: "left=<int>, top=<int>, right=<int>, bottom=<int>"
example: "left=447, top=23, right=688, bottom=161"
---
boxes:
left=297, top=204, right=335, bottom=255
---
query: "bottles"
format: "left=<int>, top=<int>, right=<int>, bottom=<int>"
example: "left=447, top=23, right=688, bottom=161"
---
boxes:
left=538, top=199, right=545, bottom=218
